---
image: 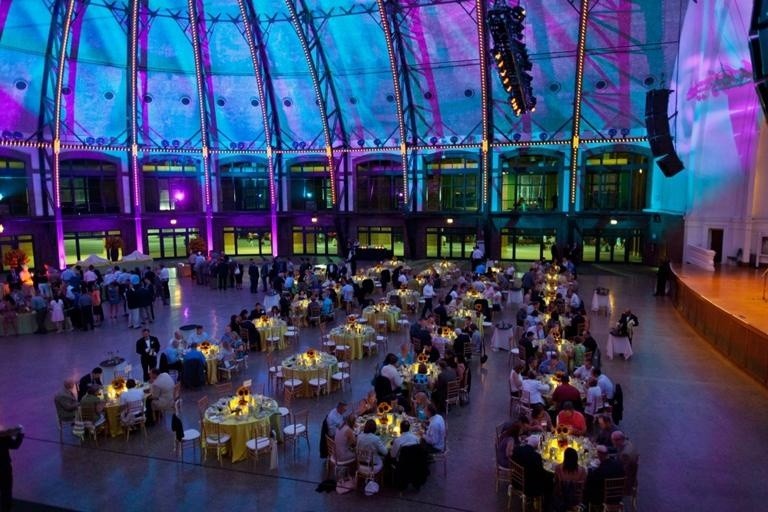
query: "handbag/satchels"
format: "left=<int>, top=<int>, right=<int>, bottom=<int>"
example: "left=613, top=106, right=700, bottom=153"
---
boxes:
left=72, top=420, right=86, bottom=437
left=335, top=475, right=358, bottom=495
left=364, top=478, right=380, bottom=497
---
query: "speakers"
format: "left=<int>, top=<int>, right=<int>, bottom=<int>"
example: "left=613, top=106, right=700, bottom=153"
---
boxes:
left=748, top=0, right=768, bottom=122
left=644, top=88, right=684, bottom=176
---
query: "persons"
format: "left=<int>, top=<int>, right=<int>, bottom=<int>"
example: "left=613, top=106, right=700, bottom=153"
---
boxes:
left=1, top=240, right=638, bottom=511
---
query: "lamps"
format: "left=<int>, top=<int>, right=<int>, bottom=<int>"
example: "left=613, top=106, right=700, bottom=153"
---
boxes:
left=483, top=1, right=537, bottom=118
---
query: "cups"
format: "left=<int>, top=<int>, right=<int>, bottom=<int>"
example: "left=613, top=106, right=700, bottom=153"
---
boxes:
left=539, top=429, right=594, bottom=465
